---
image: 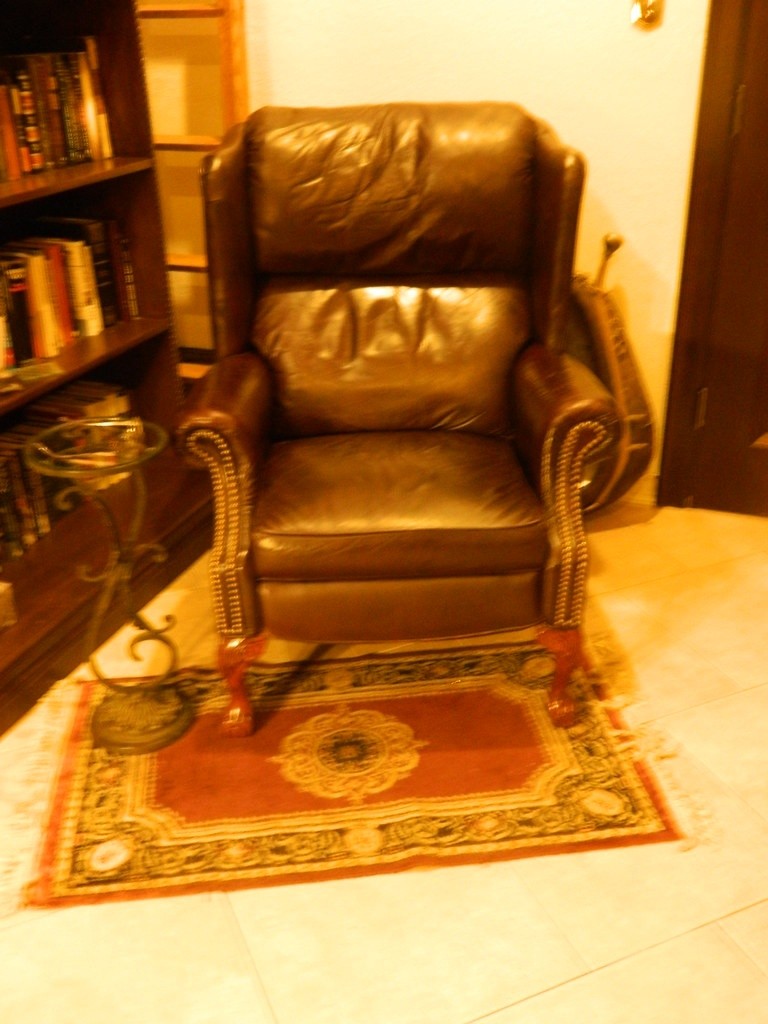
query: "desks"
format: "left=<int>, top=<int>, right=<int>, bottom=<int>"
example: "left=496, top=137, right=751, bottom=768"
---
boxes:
left=22, top=418, right=173, bottom=696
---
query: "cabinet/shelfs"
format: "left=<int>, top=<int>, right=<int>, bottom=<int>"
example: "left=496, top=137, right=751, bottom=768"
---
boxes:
left=0, top=0, right=187, bottom=662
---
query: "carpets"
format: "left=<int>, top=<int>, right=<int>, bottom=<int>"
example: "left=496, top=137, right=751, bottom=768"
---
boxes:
left=22, top=634, right=684, bottom=907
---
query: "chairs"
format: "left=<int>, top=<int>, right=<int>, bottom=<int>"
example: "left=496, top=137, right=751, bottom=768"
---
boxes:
left=170, top=108, right=656, bottom=735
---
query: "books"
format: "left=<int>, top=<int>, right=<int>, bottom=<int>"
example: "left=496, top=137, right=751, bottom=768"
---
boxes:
left=0, top=216, right=140, bottom=562
left=0, top=34, right=113, bottom=182
